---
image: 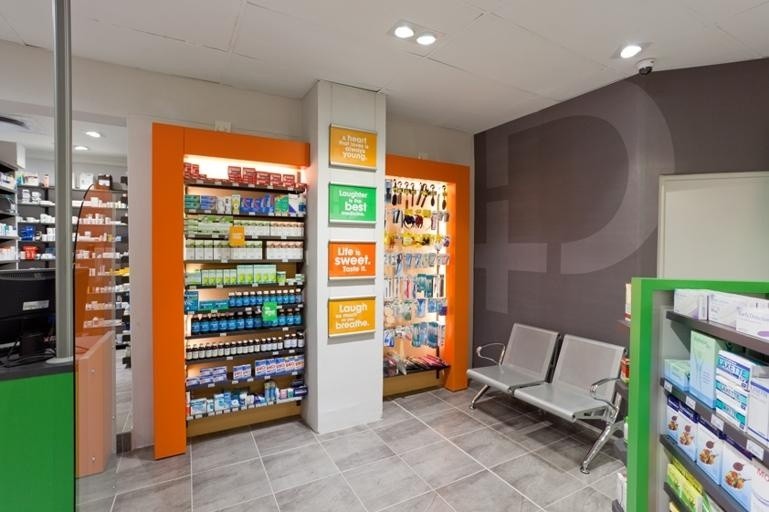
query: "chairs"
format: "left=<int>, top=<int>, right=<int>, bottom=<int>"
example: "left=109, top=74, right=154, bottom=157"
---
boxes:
left=511, top=334, right=626, bottom=474
left=466, top=322, right=561, bottom=410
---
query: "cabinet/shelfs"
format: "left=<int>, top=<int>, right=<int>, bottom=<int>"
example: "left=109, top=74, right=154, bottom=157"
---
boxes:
left=383, top=154, right=470, bottom=396
left=0, top=161, right=17, bottom=269
left=637, top=279, right=769, bottom=512
left=0, top=362, right=74, bottom=512
left=18, top=186, right=130, bottom=349
left=153, top=124, right=310, bottom=459
left=612, top=319, right=630, bottom=512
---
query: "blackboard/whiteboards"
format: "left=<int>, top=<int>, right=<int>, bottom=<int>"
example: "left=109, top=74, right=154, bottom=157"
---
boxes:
left=656, top=170, right=769, bottom=283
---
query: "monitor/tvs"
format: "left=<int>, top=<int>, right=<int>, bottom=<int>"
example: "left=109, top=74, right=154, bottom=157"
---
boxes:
left=0, top=268, right=56, bottom=368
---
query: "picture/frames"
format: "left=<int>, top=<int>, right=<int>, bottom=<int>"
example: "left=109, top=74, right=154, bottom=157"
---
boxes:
left=328, top=295, right=376, bottom=337
left=328, top=241, right=376, bottom=279
left=329, top=183, right=376, bottom=224
left=329, top=124, right=378, bottom=171
